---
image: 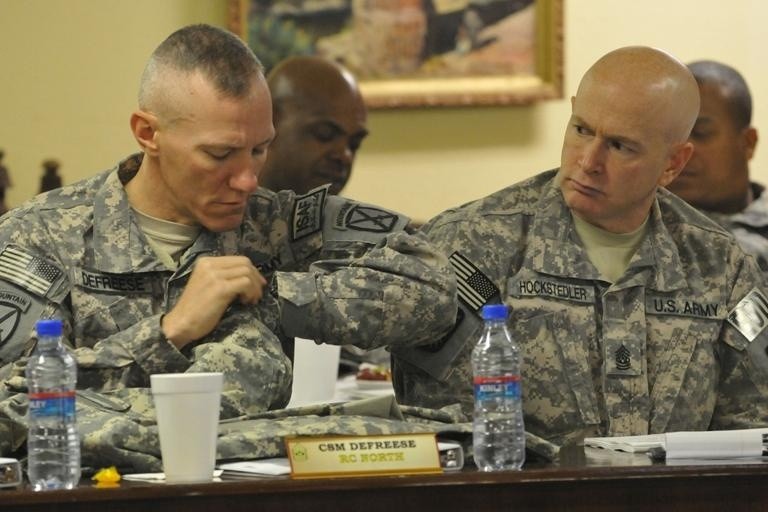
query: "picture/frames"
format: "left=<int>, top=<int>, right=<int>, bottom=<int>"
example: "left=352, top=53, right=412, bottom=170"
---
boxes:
left=223, top=0, right=569, bottom=110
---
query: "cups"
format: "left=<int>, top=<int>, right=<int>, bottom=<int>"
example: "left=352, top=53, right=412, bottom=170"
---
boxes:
left=150, top=372, right=222, bottom=485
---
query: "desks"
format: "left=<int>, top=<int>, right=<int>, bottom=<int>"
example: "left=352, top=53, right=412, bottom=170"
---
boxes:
left=3, top=443, right=767, bottom=509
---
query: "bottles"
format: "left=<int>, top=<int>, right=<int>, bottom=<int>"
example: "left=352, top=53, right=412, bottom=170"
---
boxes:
left=470, top=304, right=525, bottom=474
left=24, top=319, right=82, bottom=492
